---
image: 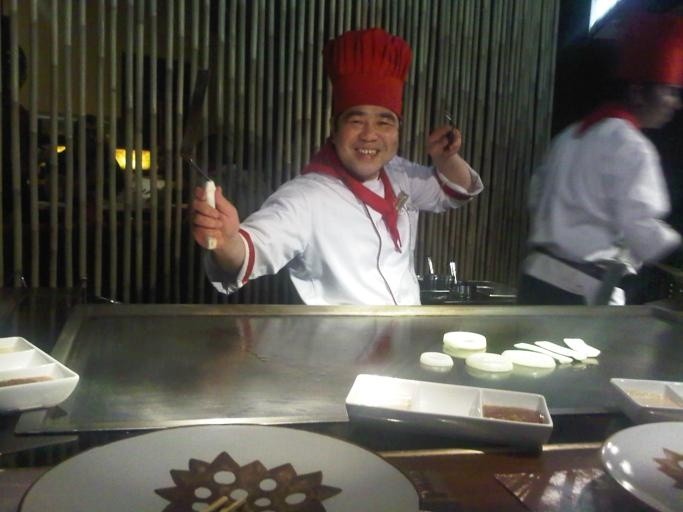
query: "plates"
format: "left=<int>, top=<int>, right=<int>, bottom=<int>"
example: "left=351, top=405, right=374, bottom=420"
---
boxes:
left=601, top=420, right=682, bottom=511
left=345, top=373, right=553, bottom=446
left=610, top=373, right=682, bottom=421
left=0, top=335, right=81, bottom=413
left=17, top=425, right=421, bottom=511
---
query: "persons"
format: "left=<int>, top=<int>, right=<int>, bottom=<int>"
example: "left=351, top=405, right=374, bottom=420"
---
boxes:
left=225, top=316, right=427, bottom=425
left=190, top=100, right=485, bottom=310
left=525, top=310, right=671, bottom=408
left=0, top=37, right=47, bottom=280
left=56, top=113, right=129, bottom=271
left=169, top=133, right=282, bottom=302
left=515, top=39, right=682, bottom=308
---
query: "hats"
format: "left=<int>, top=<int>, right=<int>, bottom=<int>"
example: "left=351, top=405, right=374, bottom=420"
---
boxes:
left=611, top=1, right=683, bottom=84
left=324, top=30, right=412, bottom=118
